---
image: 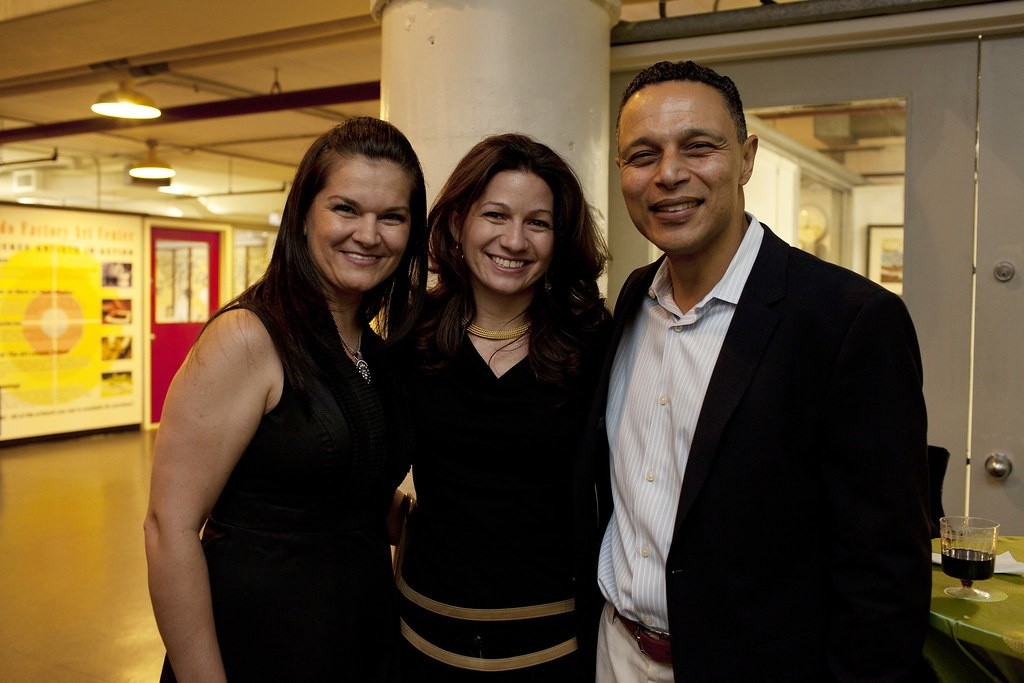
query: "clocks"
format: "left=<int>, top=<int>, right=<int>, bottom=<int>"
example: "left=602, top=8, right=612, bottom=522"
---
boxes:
left=795, top=202, right=827, bottom=244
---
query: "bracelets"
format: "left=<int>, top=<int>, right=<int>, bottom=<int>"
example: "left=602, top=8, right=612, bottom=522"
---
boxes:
left=394, top=490, right=415, bottom=546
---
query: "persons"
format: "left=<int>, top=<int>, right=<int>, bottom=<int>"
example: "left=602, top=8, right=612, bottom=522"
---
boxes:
left=592, top=61, right=931, bottom=683
left=395, top=133, right=613, bottom=683
left=144, top=116, right=428, bottom=683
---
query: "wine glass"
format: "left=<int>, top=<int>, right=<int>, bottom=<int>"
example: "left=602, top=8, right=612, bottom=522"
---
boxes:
left=940, top=516, right=1000, bottom=601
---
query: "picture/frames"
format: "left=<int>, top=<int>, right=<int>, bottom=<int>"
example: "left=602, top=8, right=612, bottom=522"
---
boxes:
left=864, top=223, right=904, bottom=296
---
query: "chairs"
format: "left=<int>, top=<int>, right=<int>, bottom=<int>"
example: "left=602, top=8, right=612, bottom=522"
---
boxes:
left=926, top=444, right=951, bottom=538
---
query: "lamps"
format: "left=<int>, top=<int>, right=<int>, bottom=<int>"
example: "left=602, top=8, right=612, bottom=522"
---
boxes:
left=127, top=140, right=175, bottom=178
left=90, top=75, right=162, bottom=119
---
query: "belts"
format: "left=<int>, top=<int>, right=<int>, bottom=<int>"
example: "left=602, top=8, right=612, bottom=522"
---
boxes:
left=614, top=606, right=673, bottom=668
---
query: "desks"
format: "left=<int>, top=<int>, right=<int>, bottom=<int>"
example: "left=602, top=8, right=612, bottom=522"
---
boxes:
left=916, top=535, right=1024, bottom=683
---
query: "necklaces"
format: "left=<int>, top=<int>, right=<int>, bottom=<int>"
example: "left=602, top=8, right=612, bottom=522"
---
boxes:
left=339, top=331, right=372, bottom=385
left=461, top=316, right=530, bottom=340
